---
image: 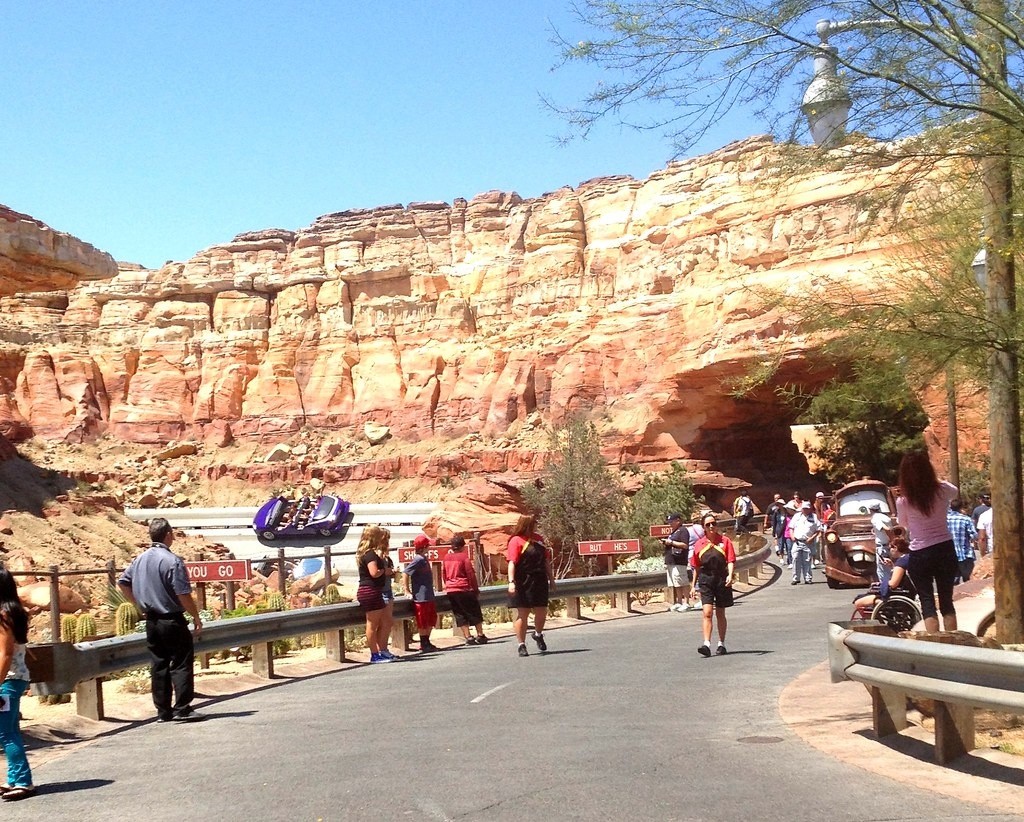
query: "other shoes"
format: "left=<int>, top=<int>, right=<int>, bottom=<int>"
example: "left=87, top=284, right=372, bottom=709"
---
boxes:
left=822, top=570, right=826, bottom=573
left=419, top=644, right=439, bottom=653
left=668, top=603, right=690, bottom=613
left=792, top=580, right=801, bottom=586
left=805, top=580, right=814, bottom=584
left=811, top=559, right=820, bottom=570
left=692, top=601, right=702, bottom=609
left=697, top=644, right=727, bottom=658
left=157, top=711, right=208, bottom=723
left=774, top=546, right=794, bottom=570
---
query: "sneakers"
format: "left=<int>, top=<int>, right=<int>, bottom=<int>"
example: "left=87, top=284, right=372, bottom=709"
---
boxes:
left=518, top=631, right=547, bottom=657
left=466, top=634, right=488, bottom=645
left=371, top=649, right=397, bottom=663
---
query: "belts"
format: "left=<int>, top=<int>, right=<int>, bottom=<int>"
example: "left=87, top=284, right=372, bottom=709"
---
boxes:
left=795, top=539, right=806, bottom=545
left=875, top=543, right=887, bottom=547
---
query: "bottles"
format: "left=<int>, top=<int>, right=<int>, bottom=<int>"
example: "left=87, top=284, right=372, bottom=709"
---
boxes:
left=394, top=566, right=401, bottom=583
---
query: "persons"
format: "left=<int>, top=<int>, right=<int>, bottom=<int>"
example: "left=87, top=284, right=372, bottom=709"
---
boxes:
left=659, top=512, right=704, bottom=612
left=854, top=501, right=911, bottom=623
left=403, top=535, right=439, bottom=651
left=507, top=512, right=558, bottom=657
left=357, top=525, right=398, bottom=663
left=896, top=450, right=959, bottom=633
left=440, top=537, right=488, bottom=643
left=946, top=499, right=975, bottom=582
left=118, top=518, right=205, bottom=720
left=762, top=491, right=833, bottom=585
left=733, top=489, right=754, bottom=534
left=689, top=514, right=736, bottom=656
left=280, top=496, right=322, bottom=527
left=971, top=491, right=993, bottom=556
left=0, top=564, right=34, bottom=800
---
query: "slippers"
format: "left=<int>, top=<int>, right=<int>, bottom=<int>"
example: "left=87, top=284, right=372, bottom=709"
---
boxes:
left=0, top=783, right=36, bottom=800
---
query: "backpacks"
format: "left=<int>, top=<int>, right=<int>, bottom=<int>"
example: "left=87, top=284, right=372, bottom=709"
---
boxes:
left=742, top=498, right=754, bottom=518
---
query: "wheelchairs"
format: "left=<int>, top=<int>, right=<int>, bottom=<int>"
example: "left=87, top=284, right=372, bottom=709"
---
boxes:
left=849, top=564, right=921, bottom=634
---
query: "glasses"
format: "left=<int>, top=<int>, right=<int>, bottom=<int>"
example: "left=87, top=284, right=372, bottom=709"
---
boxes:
left=704, top=521, right=717, bottom=527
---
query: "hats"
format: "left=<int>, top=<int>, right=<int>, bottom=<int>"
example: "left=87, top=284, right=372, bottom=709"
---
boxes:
left=413, top=536, right=431, bottom=550
left=801, top=501, right=812, bottom=510
left=867, top=502, right=881, bottom=511
left=783, top=500, right=798, bottom=512
left=816, top=492, right=824, bottom=499
left=775, top=498, right=785, bottom=505
left=665, top=512, right=680, bottom=524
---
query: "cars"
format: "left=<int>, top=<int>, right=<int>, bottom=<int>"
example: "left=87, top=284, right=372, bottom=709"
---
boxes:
left=252, top=494, right=352, bottom=541
left=825, top=476, right=909, bottom=590
